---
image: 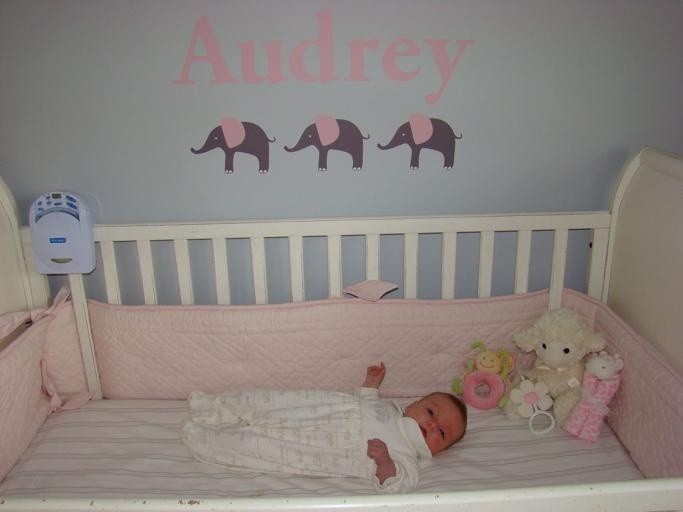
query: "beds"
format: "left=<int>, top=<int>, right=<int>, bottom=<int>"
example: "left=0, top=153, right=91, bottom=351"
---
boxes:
left=0, top=147, right=683, bottom=512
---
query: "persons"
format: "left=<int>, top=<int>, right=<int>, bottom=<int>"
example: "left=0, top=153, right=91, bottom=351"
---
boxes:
left=180, top=359, right=467, bottom=494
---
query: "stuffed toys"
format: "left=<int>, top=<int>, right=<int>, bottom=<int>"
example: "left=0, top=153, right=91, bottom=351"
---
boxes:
left=449, top=305, right=624, bottom=442
